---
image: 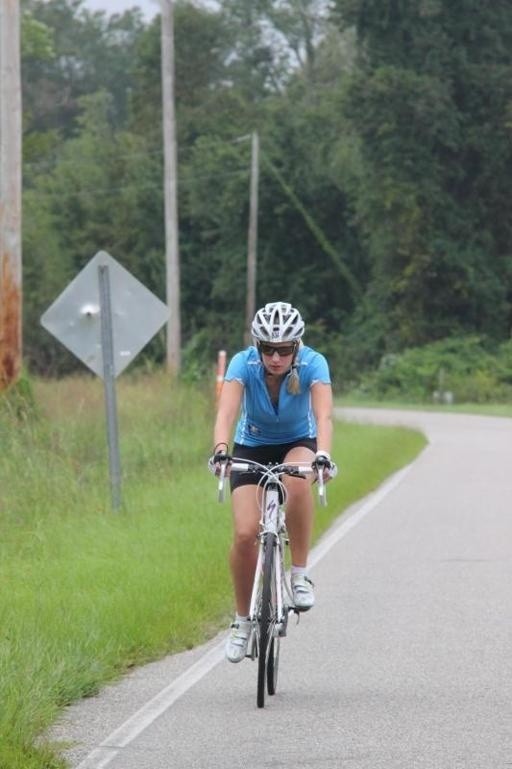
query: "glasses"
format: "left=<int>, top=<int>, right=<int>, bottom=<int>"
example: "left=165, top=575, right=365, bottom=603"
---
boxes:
left=259, top=343, right=296, bottom=356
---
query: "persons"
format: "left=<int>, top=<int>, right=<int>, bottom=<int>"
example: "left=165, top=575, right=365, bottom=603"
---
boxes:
left=212, top=299, right=334, bottom=663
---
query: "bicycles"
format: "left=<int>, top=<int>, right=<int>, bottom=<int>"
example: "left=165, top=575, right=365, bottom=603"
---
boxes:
left=203, top=449, right=335, bottom=710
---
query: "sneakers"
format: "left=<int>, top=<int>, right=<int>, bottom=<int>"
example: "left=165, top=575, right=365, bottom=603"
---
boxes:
left=290, top=575, right=315, bottom=610
left=226, top=617, right=252, bottom=663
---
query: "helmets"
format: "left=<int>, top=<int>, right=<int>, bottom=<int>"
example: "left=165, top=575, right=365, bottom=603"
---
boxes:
left=251, top=301, right=305, bottom=344
